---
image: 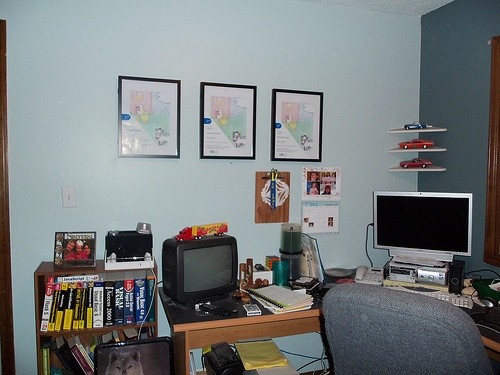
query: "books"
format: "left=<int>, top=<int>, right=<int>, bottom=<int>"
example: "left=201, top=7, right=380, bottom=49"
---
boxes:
left=40, top=268, right=155, bottom=375
left=247, top=285, right=314, bottom=316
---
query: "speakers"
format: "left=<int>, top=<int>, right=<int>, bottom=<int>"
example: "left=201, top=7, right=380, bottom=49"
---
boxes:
left=449, top=259, right=466, bottom=293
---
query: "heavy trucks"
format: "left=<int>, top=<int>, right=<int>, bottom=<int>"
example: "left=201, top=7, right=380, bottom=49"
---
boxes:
left=174, top=222, right=228, bottom=242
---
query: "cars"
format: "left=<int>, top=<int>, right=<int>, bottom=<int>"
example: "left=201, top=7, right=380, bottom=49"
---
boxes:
left=399, top=138, right=434, bottom=150
left=400, top=158, right=432, bottom=169
left=404, top=121, right=425, bottom=130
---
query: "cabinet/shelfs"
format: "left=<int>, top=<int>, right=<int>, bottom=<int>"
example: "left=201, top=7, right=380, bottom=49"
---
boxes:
left=387, top=127, right=447, bottom=171
left=34, top=259, right=158, bottom=375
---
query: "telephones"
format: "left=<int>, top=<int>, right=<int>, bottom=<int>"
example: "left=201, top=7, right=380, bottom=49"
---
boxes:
left=355, top=265, right=384, bottom=285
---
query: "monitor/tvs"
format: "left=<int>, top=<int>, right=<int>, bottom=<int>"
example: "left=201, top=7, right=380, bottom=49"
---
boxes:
left=162, top=235, right=238, bottom=307
left=372, top=191, right=473, bottom=267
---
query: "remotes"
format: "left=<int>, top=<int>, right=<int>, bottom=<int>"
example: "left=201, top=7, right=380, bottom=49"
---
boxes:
left=204, top=308, right=230, bottom=317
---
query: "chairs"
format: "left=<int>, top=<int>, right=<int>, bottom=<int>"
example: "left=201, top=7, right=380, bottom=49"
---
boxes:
left=322, top=283, right=500, bottom=375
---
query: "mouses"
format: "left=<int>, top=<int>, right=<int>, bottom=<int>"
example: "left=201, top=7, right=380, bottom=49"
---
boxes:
left=481, top=296, right=497, bottom=307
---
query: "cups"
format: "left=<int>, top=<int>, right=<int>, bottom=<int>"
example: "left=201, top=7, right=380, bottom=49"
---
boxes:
left=272, top=260, right=289, bottom=286
left=279, top=223, right=303, bottom=281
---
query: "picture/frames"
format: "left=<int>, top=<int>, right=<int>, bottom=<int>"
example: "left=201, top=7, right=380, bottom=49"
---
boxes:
left=54, top=231, right=97, bottom=268
left=271, top=89, right=324, bottom=162
left=118, top=75, right=180, bottom=158
left=200, top=82, right=257, bottom=160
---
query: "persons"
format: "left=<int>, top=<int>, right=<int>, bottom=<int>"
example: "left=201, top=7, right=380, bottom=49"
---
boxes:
left=64, top=241, right=91, bottom=261
left=308, top=172, right=337, bottom=195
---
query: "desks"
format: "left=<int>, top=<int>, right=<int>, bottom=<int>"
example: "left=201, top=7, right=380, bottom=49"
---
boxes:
left=157, top=286, right=500, bottom=375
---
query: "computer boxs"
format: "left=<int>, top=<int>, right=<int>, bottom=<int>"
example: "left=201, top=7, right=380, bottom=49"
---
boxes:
left=382, top=260, right=449, bottom=286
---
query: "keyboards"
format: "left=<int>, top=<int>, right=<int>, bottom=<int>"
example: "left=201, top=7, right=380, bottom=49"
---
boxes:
left=385, top=284, right=473, bottom=310
